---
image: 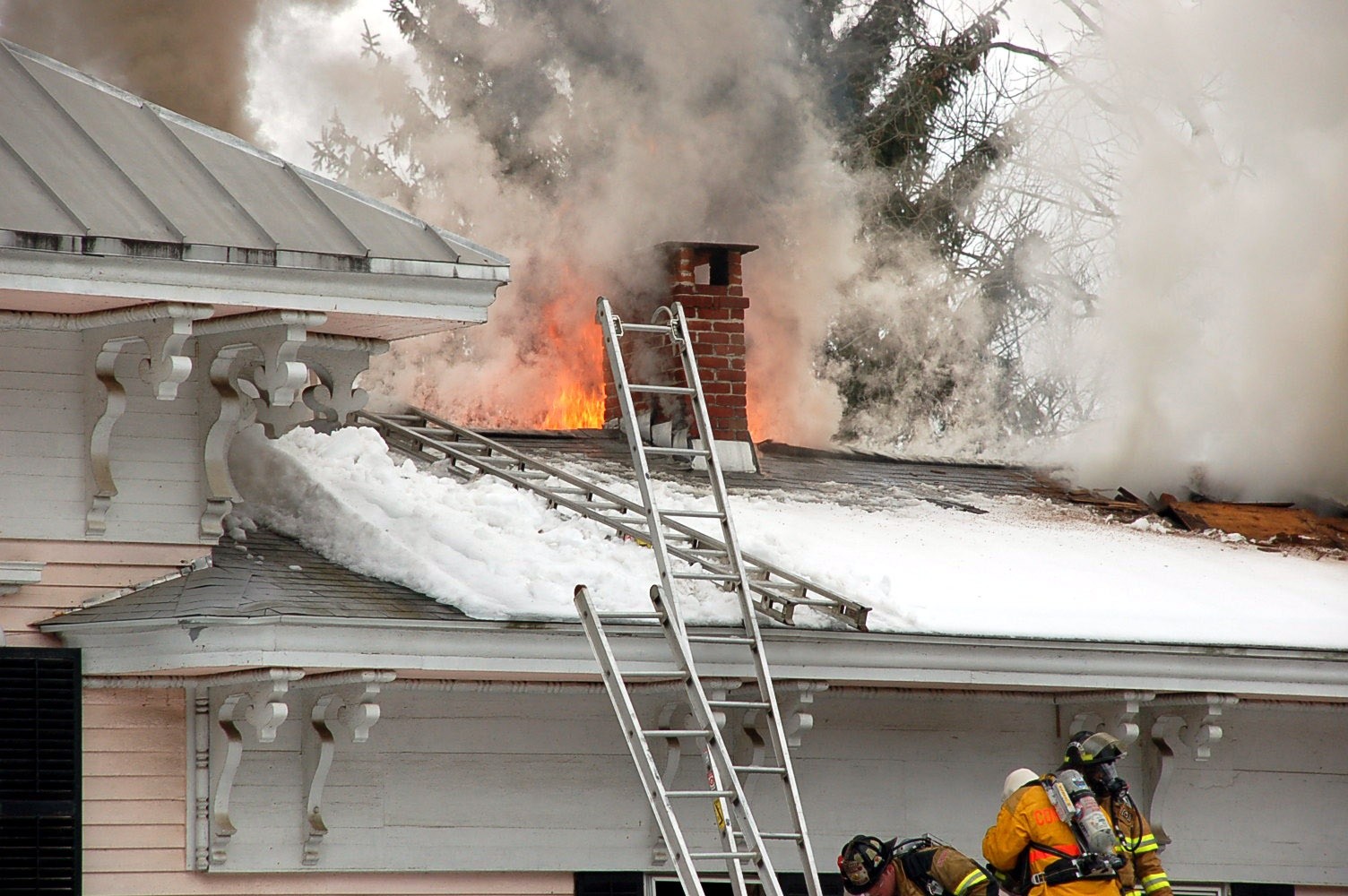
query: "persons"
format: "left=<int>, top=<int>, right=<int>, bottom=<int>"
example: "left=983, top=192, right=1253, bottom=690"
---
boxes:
left=837, top=833, right=1000, bottom=896
left=1055, top=730, right=1173, bottom=896
left=983, top=768, right=1126, bottom=896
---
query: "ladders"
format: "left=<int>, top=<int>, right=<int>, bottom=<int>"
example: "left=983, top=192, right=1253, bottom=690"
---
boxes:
left=572, top=583, right=788, bottom=896
left=597, top=295, right=821, bottom=896
left=347, top=404, right=872, bottom=631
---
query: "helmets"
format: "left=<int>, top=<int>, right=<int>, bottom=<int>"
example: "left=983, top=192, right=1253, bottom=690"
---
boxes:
left=837, top=835, right=898, bottom=895
left=1002, top=768, right=1039, bottom=801
left=1055, top=733, right=1122, bottom=771
